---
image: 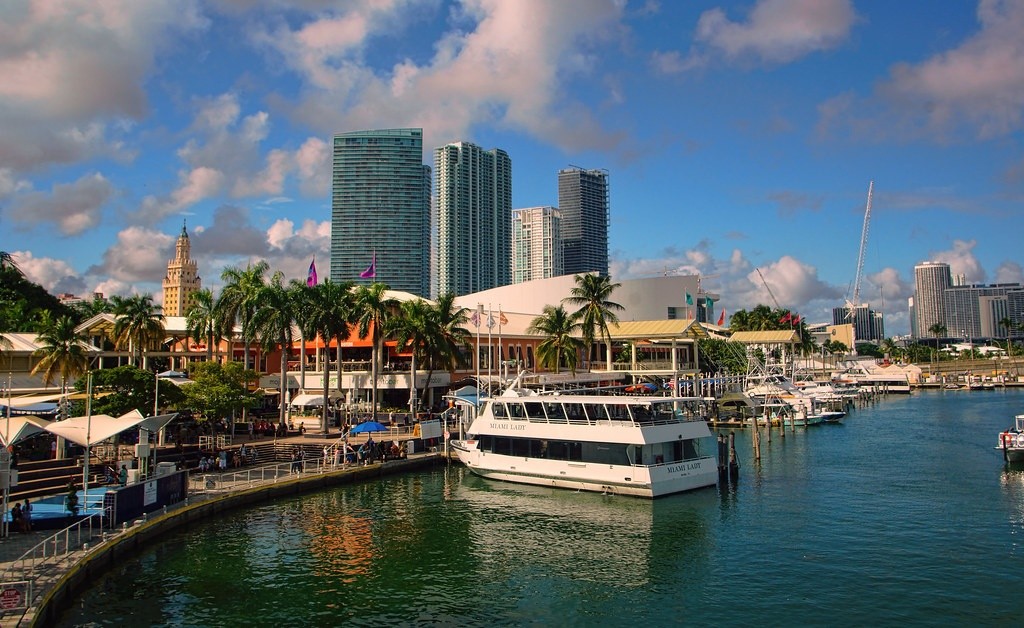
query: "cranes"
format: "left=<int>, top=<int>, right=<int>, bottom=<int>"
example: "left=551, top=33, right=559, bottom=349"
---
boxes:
left=844, top=180, right=874, bottom=356
left=641, top=265, right=678, bottom=277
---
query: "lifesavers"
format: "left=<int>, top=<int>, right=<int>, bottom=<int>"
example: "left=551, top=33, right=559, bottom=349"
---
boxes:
left=626, top=385, right=650, bottom=392
left=1001, top=433, right=1011, bottom=444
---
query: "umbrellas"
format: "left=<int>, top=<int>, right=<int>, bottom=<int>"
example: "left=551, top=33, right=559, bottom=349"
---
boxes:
left=350, top=421, right=387, bottom=439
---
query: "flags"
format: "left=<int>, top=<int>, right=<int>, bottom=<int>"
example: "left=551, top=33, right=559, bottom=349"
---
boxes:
left=685, top=292, right=693, bottom=305
left=706, top=297, right=713, bottom=308
left=307, top=260, right=317, bottom=287
left=779, top=312, right=791, bottom=322
left=500, top=310, right=508, bottom=325
left=471, top=312, right=481, bottom=326
left=793, top=315, right=800, bottom=326
left=717, top=310, right=725, bottom=325
left=361, top=254, right=376, bottom=278
left=486, top=314, right=496, bottom=329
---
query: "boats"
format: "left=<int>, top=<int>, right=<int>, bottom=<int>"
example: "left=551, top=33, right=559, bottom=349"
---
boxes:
left=704, top=348, right=912, bottom=429
left=994, top=414, right=1024, bottom=456
left=450, top=370, right=720, bottom=501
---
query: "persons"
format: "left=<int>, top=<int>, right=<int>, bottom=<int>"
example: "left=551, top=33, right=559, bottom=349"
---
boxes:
left=292, top=446, right=305, bottom=473
left=198, top=443, right=258, bottom=473
left=388, top=410, right=456, bottom=433
left=323, top=437, right=407, bottom=468
left=248, top=418, right=306, bottom=440
left=118, top=464, right=128, bottom=486
left=340, top=414, right=374, bottom=440
left=385, top=359, right=404, bottom=371
left=11, top=498, right=32, bottom=534
